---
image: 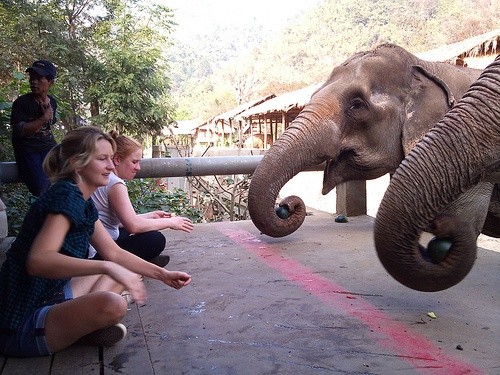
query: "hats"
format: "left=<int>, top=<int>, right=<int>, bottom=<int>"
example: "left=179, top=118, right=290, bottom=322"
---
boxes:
left=26, top=60, right=56, bottom=80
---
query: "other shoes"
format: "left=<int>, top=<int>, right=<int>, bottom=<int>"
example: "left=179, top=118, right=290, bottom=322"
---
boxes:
left=121, top=289, right=133, bottom=304
left=75, top=322, right=127, bottom=346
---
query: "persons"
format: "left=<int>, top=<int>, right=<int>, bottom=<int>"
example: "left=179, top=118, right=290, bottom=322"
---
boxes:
left=0, top=126, right=192, bottom=359
left=10, top=60, right=58, bottom=198
left=88, top=130, right=194, bottom=270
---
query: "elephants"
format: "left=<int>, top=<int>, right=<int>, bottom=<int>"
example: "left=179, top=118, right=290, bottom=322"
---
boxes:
left=374, top=54, right=500, bottom=293
left=248, top=44, right=485, bottom=238
